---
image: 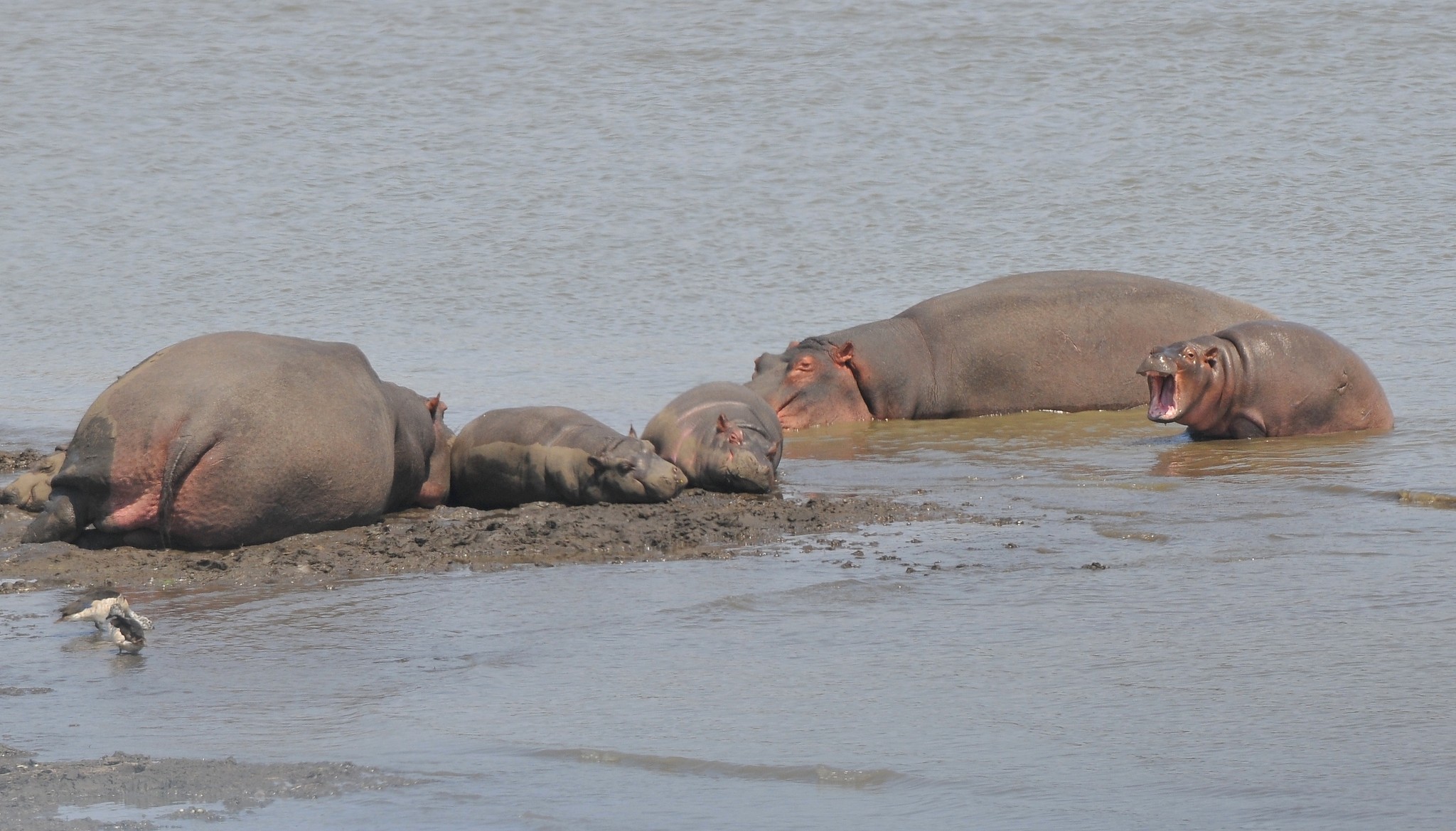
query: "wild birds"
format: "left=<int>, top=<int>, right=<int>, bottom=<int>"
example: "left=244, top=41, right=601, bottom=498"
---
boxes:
left=53, top=589, right=154, bottom=634
left=105, top=603, right=147, bottom=655
